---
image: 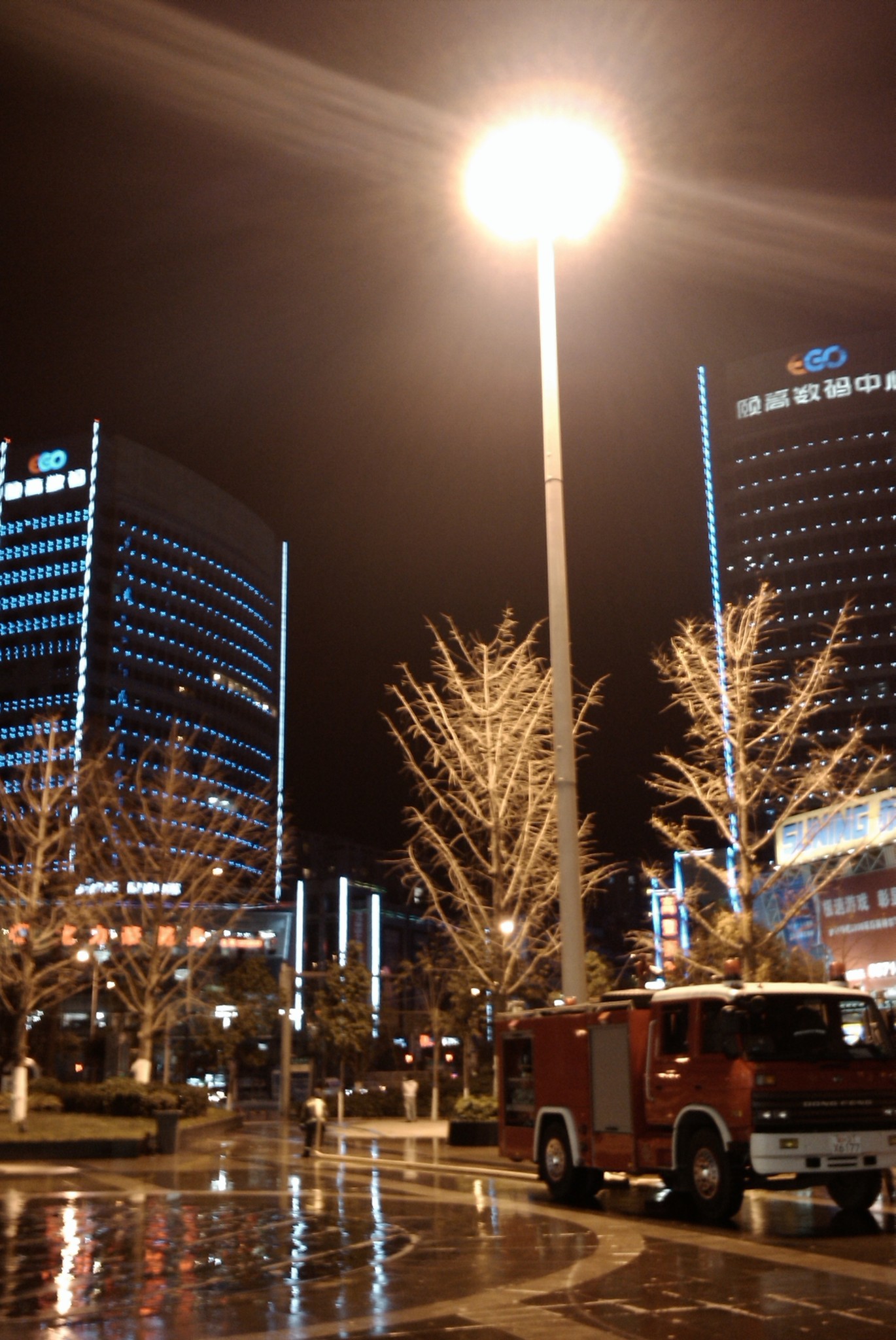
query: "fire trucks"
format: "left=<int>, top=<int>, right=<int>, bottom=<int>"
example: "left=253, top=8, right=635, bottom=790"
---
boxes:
left=490, top=976, right=895, bottom=1225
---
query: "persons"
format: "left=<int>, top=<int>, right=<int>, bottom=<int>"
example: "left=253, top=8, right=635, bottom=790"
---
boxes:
left=301, top=1088, right=327, bottom=1157
left=130, top=1049, right=152, bottom=1086
left=3, top=1044, right=39, bottom=1079
left=401, top=1073, right=418, bottom=1123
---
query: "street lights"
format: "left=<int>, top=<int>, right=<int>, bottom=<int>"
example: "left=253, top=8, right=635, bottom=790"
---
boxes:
left=186, top=863, right=224, bottom=1013
left=452, top=102, right=629, bottom=1004
